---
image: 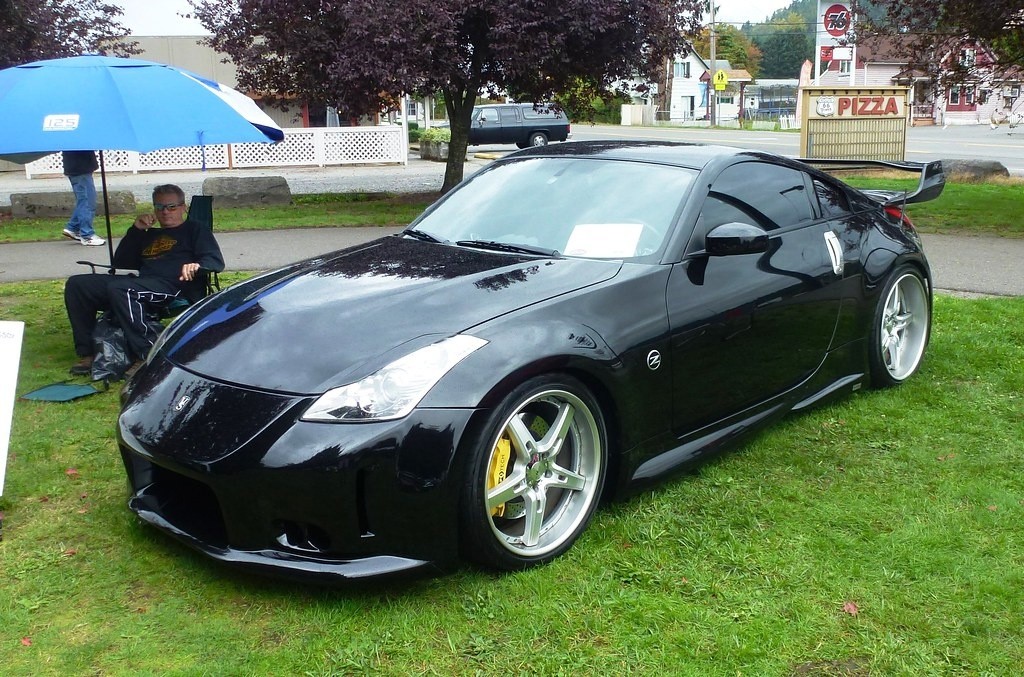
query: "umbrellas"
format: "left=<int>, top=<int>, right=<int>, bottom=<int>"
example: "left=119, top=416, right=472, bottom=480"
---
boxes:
left=0, top=56, right=284, bottom=275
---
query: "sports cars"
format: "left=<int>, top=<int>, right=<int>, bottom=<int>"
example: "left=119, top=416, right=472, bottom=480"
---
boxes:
left=111, top=139, right=944, bottom=590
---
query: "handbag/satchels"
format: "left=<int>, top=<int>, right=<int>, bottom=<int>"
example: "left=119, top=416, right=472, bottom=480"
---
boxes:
left=90, top=317, right=136, bottom=390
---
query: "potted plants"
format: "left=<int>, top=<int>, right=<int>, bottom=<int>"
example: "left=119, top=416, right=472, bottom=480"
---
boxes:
left=418, top=128, right=451, bottom=162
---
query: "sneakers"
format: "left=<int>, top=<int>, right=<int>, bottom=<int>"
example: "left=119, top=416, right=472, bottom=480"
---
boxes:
left=81, top=234, right=106, bottom=246
left=62, top=229, right=81, bottom=240
left=125, top=360, right=144, bottom=376
left=69, top=356, right=94, bottom=374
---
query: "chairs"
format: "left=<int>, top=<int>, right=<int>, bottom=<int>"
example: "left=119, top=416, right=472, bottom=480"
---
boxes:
left=77, top=196, right=220, bottom=324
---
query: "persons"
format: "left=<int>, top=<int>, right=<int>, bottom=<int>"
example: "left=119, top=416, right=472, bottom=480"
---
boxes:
left=65, top=185, right=225, bottom=376
left=62, top=150, right=107, bottom=246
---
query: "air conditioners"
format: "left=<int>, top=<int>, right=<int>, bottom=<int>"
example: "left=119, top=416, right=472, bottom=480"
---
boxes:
left=1010, top=89, right=1019, bottom=98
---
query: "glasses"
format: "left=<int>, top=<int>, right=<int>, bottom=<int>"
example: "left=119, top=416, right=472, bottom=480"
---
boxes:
left=153, top=203, right=182, bottom=211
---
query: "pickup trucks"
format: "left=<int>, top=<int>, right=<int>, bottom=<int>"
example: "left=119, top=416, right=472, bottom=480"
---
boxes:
left=467, top=102, right=571, bottom=149
